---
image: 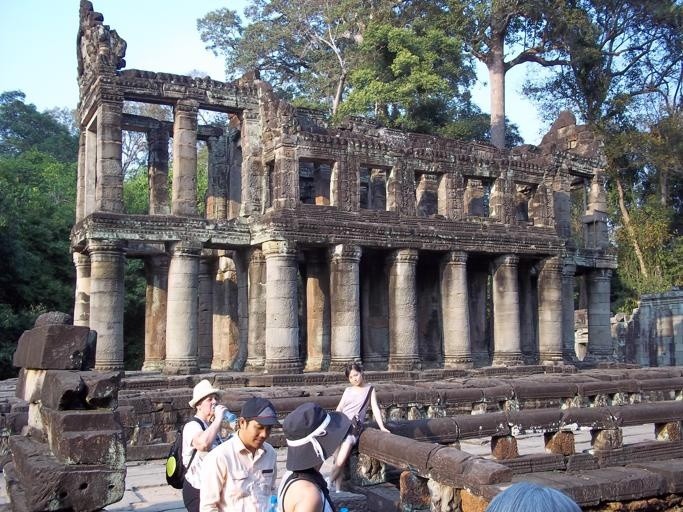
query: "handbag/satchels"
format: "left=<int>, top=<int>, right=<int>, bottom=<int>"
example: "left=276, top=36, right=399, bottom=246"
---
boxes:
left=351, top=416, right=362, bottom=437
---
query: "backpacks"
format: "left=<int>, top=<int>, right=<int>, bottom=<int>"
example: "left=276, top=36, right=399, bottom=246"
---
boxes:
left=166, top=417, right=221, bottom=489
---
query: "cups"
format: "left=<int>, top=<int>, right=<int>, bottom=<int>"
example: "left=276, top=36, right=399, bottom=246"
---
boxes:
left=211, top=404, right=236, bottom=422
left=267, top=494, right=278, bottom=511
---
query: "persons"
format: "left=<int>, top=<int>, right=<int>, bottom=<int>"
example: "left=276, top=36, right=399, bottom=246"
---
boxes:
left=277, top=403, right=352, bottom=512
left=324, top=365, right=392, bottom=493
left=199, top=397, right=278, bottom=512
left=484, top=482, right=583, bottom=512
left=181, top=379, right=228, bottom=512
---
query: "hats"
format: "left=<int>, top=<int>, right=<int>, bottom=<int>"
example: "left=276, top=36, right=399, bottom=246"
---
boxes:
left=283, top=403, right=351, bottom=471
left=188, top=380, right=223, bottom=409
left=241, top=397, right=279, bottom=426
